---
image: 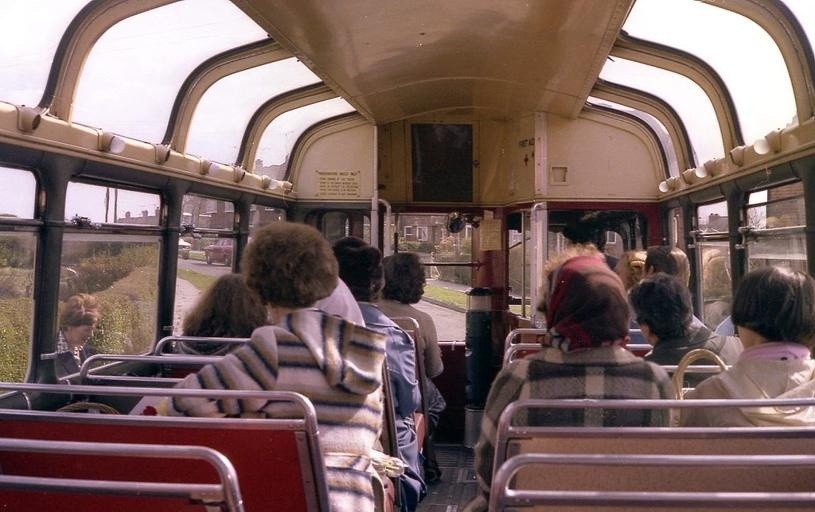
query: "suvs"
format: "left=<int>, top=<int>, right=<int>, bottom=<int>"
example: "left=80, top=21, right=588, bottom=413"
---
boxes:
left=203, top=237, right=252, bottom=266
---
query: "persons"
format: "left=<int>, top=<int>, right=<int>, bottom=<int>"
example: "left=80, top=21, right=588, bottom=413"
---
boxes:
left=167, top=220, right=444, bottom=512
left=466, top=219, right=815, bottom=511
left=53, top=291, right=103, bottom=378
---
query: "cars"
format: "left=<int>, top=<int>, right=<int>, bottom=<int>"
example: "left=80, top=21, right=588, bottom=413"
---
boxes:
left=177, top=238, right=192, bottom=259
